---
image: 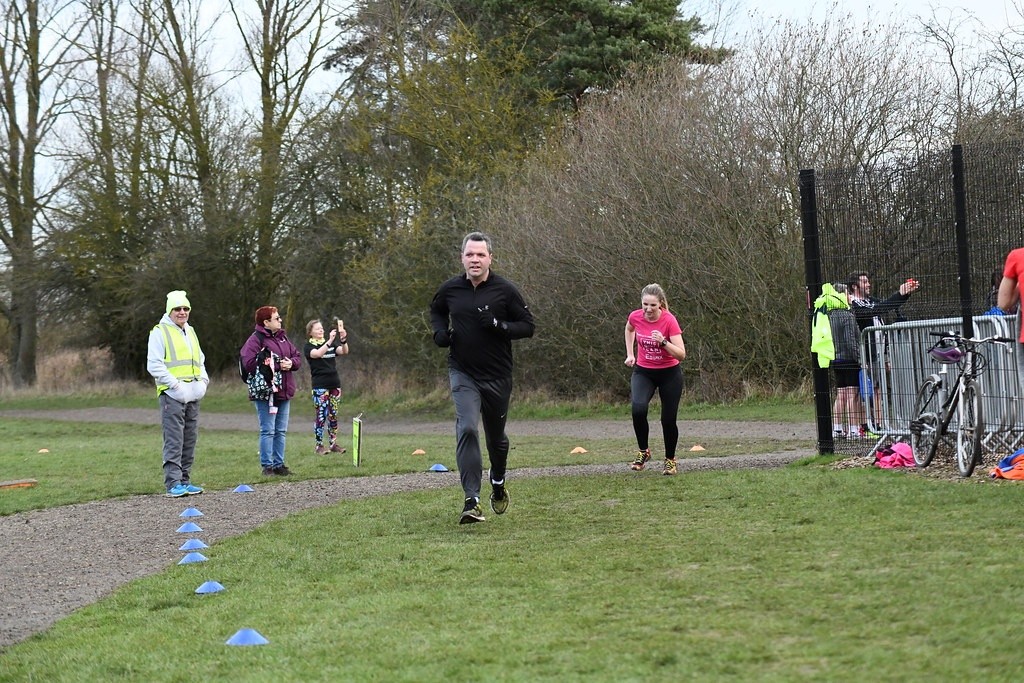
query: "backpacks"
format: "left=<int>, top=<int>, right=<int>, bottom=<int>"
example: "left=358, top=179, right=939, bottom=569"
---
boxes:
left=238, top=331, right=265, bottom=383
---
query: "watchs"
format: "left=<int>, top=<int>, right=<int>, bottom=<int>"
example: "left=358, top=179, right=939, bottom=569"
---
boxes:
left=661, top=339, right=667, bottom=347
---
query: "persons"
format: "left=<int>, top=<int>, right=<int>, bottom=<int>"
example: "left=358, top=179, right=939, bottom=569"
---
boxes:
left=146, top=290, right=210, bottom=497
left=429, top=233, right=535, bottom=524
left=624, top=284, right=686, bottom=474
left=240, top=307, right=301, bottom=476
left=983, top=247, right=1024, bottom=344
left=304, top=319, right=349, bottom=455
left=830, top=271, right=920, bottom=439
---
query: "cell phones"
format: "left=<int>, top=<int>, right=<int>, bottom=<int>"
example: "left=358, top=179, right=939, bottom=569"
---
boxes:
left=337, top=319, right=343, bottom=332
left=906, top=278, right=914, bottom=288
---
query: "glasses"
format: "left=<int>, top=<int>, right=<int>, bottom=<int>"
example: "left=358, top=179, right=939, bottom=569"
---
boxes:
left=172, top=307, right=190, bottom=312
left=267, top=316, right=282, bottom=322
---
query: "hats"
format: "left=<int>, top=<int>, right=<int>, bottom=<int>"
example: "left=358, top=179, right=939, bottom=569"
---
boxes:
left=166, top=290, right=192, bottom=316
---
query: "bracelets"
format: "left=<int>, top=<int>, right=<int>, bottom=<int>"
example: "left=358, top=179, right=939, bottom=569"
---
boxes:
left=341, top=339, right=347, bottom=344
left=326, top=344, right=330, bottom=348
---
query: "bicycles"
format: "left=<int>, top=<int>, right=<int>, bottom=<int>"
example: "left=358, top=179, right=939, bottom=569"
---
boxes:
left=909, top=331, right=1016, bottom=478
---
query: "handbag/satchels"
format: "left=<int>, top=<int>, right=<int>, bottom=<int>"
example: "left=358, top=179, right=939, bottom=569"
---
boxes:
left=245, top=350, right=282, bottom=401
left=989, top=446, right=1024, bottom=482
left=872, top=442, right=917, bottom=469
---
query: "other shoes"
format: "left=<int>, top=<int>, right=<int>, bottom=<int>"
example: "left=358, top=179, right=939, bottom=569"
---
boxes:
left=847, top=430, right=867, bottom=441
left=831, top=429, right=848, bottom=440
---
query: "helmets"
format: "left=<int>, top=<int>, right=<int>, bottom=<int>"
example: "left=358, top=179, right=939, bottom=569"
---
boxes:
left=927, top=340, right=965, bottom=364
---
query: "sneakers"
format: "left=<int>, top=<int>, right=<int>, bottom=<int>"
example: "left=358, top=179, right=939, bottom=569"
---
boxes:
left=631, top=448, right=651, bottom=471
left=858, top=422, right=882, bottom=440
left=182, top=484, right=203, bottom=495
left=273, top=464, right=293, bottom=476
left=330, top=444, right=346, bottom=454
left=459, top=498, right=486, bottom=523
left=488, top=468, right=509, bottom=515
left=662, top=457, right=677, bottom=475
left=315, top=444, right=329, bottom=455
left=166, top=484, right=189, bottom=498
left=262, top=467, right=276, bottom=476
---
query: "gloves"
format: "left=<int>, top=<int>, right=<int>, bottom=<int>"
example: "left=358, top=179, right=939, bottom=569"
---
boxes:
left=433, top=329, right=451, bottom=348
left=479, top=309, right=502, bottom=332
